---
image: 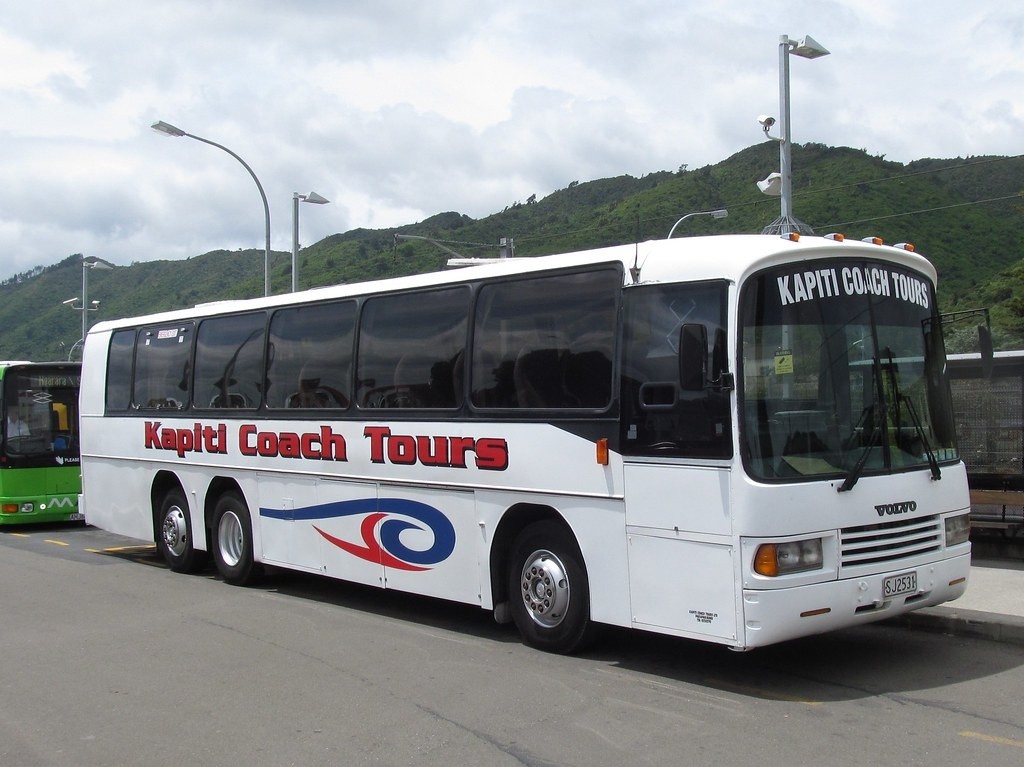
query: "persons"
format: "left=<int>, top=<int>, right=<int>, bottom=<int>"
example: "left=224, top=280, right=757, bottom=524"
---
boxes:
left=7, top=404, right=30, bottom=437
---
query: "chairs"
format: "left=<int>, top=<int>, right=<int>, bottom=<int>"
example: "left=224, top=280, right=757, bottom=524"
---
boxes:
left=130, top=333, right=614, bottom=407
left=965, top=466, right=1024, bottom=542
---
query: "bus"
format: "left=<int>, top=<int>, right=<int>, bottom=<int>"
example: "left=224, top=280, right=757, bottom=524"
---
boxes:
left=77, top=232, right=995, bottom=655
left=0, top=360, right=85, bottom=526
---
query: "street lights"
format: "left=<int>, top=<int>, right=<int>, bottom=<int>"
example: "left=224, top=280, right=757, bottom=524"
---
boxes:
left=150, top=120, right=271, bottom=298
left=292, top=191, right=330, bottom=293
left=667, top=209, right=729, bottom=238
left=82, top=261, right=113, bottom=346
left=757, top=34, right=832, bottom=398
